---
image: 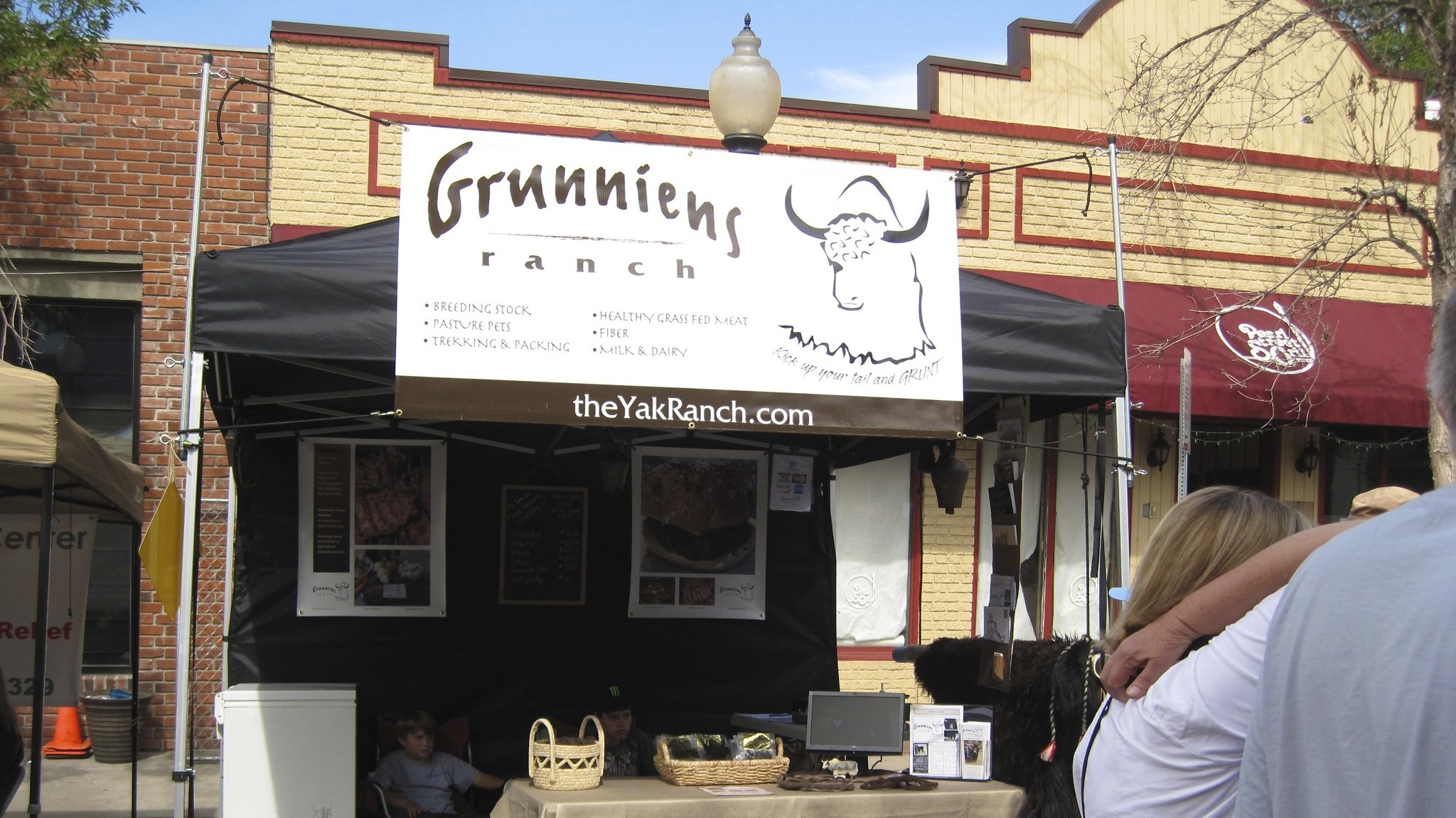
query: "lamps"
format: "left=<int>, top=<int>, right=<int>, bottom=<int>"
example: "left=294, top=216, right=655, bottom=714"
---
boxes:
left=955, top=159, right=973, bottom=209
left=708, top=13, right=781, bottom=155
left=1146, top=426, right=1172, bottom=472
left=1295, top=433, right=1321, bottom=478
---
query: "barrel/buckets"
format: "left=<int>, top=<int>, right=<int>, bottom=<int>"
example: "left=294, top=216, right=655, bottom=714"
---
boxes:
left=79, top=689, right=155, bottom=764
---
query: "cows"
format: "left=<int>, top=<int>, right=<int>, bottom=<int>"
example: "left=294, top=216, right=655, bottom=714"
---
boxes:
left=821, top=758, right=859, bottom=780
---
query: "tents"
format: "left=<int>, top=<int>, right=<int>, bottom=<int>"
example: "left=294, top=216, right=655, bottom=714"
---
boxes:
left=0, top=360, right=144, bottom=818
left=168, top=214, right=1128, bottom=818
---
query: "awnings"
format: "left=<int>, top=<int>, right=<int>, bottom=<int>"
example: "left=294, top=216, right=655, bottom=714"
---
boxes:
left=971, top=269, right=1436, bottom=430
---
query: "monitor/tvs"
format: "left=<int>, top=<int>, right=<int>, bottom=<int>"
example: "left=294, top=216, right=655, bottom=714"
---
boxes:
left=806, top=691, right=905, bottom=777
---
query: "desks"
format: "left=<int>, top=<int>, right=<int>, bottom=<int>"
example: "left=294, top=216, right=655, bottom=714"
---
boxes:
left=488, top=777, right=1029, bottom=818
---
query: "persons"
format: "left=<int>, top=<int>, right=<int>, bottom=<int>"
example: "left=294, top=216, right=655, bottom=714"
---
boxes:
left=1347, top=487, right=1421, bottom=520
left=1072, top=486, right=1373, bottom=818
left=583, top=686, right=660, bottom=778
left=0, top=669, right=25, bottom=818
left=359, top=710, right=508, bottom=818
left=1235, top=285, right=1456, bottom=818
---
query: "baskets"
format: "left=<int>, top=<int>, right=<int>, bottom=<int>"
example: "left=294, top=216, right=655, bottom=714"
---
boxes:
left=529, top=715, right=605, bottom=790
left=653, top=737, right=790, bottom=787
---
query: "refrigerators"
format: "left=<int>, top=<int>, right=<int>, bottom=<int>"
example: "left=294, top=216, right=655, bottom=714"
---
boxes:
left=214, top=682, right=357, bottom=818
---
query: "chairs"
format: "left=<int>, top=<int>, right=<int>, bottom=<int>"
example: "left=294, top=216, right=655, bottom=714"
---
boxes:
left=357, top=715, right=475, bottom=818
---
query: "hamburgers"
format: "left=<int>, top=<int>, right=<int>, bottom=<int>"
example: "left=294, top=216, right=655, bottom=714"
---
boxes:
left=640, top=462, right=755, bottom=572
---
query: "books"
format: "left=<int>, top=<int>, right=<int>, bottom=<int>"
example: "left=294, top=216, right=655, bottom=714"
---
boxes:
left=984, top=398, right=1026, bottom=646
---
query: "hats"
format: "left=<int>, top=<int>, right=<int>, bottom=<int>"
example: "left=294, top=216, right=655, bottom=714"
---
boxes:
left=1347, top=486, right=1420, bottom=519
left=595, top=684, right=631, bottom=715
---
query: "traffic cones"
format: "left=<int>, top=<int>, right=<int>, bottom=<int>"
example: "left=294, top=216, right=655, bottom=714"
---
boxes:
left=45, top=705, right=90, bottom=752
